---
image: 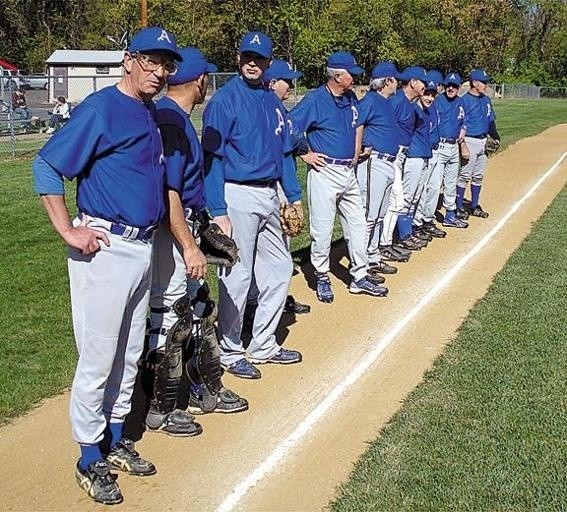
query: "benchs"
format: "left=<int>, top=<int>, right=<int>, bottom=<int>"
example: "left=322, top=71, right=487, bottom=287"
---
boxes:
left=0, top=112, right=32, bottom=135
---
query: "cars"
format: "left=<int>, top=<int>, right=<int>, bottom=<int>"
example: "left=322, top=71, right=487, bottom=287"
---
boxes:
left=0, top=68, right=48, bottom=89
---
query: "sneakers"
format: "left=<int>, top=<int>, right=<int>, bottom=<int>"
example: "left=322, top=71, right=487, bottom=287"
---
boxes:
left=442, top=205, right=490, bottom=228
left=246, top=348, right=302, bottom=364
left=283, top=296, right=311, bottom=316
left=74, top=455, right=124, bottom=505
left=219, top=358, right=262, bottom=380
left=187, top=383, right=250, bottom=415
left=107, top=436, right=157, bottom=477
left=145, top=408, right=203, bottom=438
left=314, top=270, right=335, bottom=304
left=379, top=220, right=447, bottom=263
left=348, top=261, right=399, bottom=297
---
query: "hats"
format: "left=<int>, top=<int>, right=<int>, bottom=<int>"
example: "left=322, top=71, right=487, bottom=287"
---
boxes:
left=261, top=59, right=305, bottom=84
left=166, top=46, right=218, bottom=85
left=127, top=27, right=183, bottom=62
left=326, top=49, right=367, bottom=78
left=371, top=61, right=493, bottom=94
left=238, top=31, right=273, bottom=61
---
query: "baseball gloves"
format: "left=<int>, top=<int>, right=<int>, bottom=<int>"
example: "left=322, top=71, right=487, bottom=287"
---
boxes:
left=201, top=224, right=237, bottom=267
left=484, top=137, right=500, bottom=159
left=280, top=201, right=303, bottom=237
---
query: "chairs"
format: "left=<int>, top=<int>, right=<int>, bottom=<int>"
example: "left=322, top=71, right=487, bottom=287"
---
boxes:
left=55, top=102, right=71, bottom=131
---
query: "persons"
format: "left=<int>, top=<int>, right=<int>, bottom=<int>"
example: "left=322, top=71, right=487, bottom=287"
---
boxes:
left=12, top=89, right=32, bottom=134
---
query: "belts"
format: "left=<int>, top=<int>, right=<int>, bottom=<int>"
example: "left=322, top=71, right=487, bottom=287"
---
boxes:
left=376, top=152, right=398, bottom=161
left=222, top=177, right=284, bottom=189
left=322, top=156, right=356, bottom=167
left=466, top=134, right=489, bottom=139
left=75, top=211, right=161, bottom=241
left=439, top=138, right=458, bottom=145
